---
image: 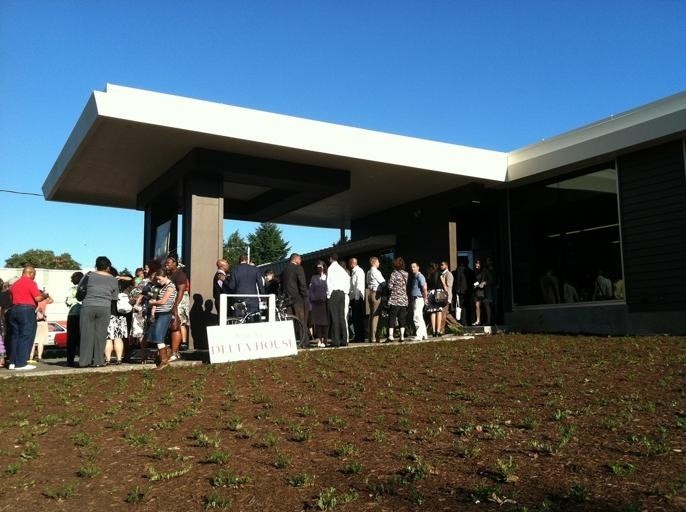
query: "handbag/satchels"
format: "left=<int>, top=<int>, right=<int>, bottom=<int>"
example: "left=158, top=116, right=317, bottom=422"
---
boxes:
left=433, top=290, right=448, bottom=307
left=75, top=279, right=88, bottom=302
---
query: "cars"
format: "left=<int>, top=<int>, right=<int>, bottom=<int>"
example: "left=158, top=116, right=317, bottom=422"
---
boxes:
left=33, top=320, right=67, bottom=347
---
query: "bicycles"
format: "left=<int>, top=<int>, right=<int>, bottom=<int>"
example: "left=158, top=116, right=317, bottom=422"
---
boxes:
left=225, top=291, right=307, bottom=348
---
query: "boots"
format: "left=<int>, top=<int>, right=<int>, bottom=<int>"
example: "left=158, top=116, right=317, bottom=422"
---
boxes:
left=152, top=347, right=170, bottom=370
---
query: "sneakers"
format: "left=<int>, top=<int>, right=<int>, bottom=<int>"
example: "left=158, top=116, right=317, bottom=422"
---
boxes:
left=168, top=353, right=182, bottom=361
left=301, top=332, right=444, bottom=348
left=8, top=363, right=15, bottom=370
left=14, top=364, right=37, bottom=371
left=102, top=360, right=123, bottom=366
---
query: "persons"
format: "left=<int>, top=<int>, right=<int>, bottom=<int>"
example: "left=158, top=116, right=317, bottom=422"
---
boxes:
left=66, top=252, right=189, bottom=368
left=1, top=265, right=53, bottom=368
left=211, top=250, right=490, bottom=348
left=521, top=268, right=626, bottom=302
